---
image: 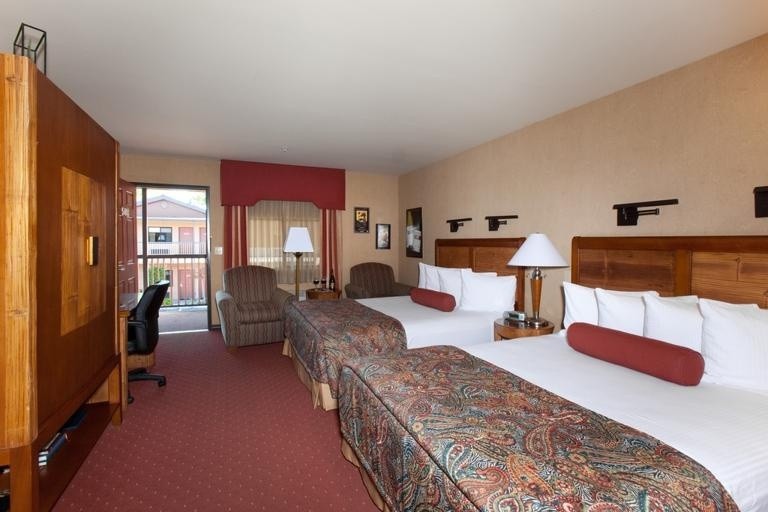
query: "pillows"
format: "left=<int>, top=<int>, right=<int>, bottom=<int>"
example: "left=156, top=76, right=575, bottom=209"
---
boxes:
left=462, top=273, right=517, bottom=312
left=438, top=267, right=498, bottom=297
left=697, top=297, right=767, bottom=391
left=562, top=283, right=659, bottom=330
left=643, top=294, right=758, bottom=355
left=418, top=263, right=426, bottom=290
left=567, top=321, right=704, bottom=387
left=425, top=266, right=471, bottom=292
left=594, top=288, right=698, bottom=337
left=410, top=287, right=456, bottom=313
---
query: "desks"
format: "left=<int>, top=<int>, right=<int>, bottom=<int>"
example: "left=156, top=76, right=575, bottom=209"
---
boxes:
left=120, top=292, right=144, bottom=410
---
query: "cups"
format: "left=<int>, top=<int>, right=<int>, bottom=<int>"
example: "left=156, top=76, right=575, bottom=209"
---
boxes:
left=412, top=230, right=421, bottom=253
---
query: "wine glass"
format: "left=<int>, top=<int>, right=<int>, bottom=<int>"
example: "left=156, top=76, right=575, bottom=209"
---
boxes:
left=313, top=280, right=319, bottom=292
left=321, top=279, right=326, bottom=290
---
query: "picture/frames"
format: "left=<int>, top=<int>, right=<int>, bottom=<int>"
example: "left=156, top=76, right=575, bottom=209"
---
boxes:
left=354, top=207, right=369, bottom=234
left=405, top=207, right=422, bottom=258
left=375, top=224, right=391, bottom=249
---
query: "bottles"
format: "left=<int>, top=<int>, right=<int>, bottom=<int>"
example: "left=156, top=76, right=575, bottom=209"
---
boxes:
left=406, top=210, right=413, bottom=251
left=329, top=274, right=335, bottom=292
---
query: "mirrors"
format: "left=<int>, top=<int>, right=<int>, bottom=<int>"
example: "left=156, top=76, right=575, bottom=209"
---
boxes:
left=339, top=237, right=768, bottom=512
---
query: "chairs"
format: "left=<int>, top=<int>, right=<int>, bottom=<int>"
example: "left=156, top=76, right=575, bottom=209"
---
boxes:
left=214, top=266, right=294, bottom=348
left=345, top=263, right=417, bottom=299
left=126, top=280, right=170, bottom=402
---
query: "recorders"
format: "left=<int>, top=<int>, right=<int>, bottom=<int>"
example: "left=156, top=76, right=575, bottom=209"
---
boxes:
left=502, top=309, right=527, bottom=322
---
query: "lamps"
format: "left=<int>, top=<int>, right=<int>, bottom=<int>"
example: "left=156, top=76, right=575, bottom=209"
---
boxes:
left=283, top=227, right=314, bottom=302
left=507, top=234, right=569, bottom=327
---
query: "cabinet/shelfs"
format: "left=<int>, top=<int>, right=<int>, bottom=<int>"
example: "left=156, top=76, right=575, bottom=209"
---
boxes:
left=1, top=54, right=120, bottom=511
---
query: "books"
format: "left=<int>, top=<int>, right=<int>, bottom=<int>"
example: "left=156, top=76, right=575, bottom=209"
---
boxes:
left=37, top=408, right=89, bottom=467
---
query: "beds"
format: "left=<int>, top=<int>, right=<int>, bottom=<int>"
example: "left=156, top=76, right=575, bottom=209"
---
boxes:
left=282, top=239, right=527, bottom=411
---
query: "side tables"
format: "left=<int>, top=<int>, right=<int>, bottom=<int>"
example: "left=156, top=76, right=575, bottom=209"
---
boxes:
left=494, top=317, right=555, bottom=341
left=306, top=288, right=342, bottom=301
